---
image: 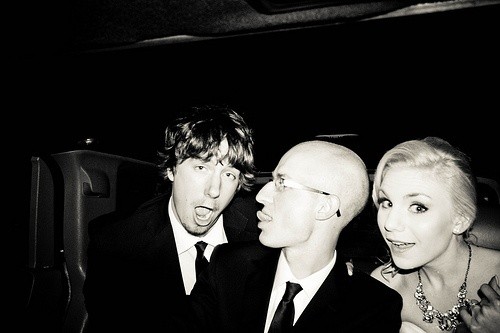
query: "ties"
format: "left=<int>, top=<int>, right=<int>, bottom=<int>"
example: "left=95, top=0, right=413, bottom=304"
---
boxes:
left=267, top=281, right=302, bottom=333
left=194, top=241, right=209, bottom=284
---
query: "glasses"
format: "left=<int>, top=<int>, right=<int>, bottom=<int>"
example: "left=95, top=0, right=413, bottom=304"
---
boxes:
left=269, top=178, right=341, bottom=218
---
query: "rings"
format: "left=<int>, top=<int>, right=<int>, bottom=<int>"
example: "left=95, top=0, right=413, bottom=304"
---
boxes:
left=468, top=302, right=482, bottom=312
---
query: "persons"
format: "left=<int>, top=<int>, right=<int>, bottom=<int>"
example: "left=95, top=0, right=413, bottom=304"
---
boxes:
left=177, top=140, right=403, bottom=333
left=368, top=138, right=500, bottom=333
left=84, top=103, right=354, bottom=333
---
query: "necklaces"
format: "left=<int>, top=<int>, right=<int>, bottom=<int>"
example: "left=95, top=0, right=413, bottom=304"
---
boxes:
left=413, top=239, right=472, bottom=331
